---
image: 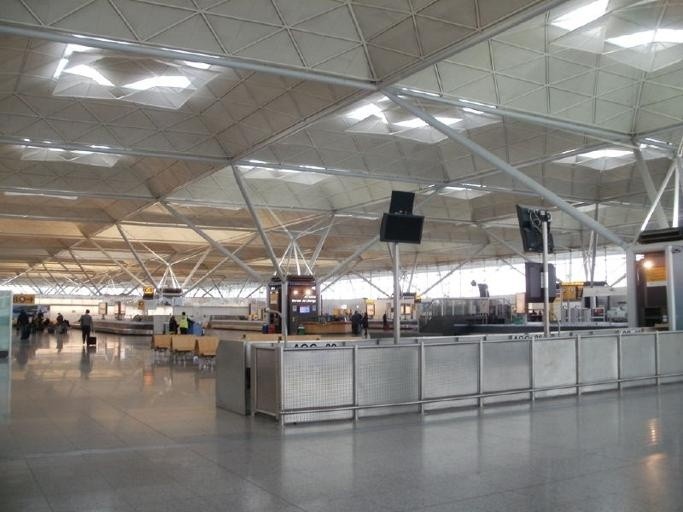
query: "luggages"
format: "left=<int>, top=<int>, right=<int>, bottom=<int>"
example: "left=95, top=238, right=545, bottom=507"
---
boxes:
left=87, top=328, right=97, bottom=346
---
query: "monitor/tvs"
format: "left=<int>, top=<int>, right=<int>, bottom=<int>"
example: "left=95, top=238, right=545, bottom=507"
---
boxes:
left=525, top=262, right=556, bottom=303
left=380, top=212, right=425, bottom=244
left=516, top=204, right=554, bottom=253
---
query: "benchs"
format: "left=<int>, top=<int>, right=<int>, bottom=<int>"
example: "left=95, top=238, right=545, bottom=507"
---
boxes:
left=150, top=333, right=221, bottom=370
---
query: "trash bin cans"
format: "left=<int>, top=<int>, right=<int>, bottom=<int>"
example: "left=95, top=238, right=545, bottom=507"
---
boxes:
left=298, top=324, right=305, bottom=335
left=454, top=324, right=469, bottom=335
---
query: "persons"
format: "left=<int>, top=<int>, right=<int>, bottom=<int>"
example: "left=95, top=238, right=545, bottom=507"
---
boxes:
left=16, top=308, right=70, bottom=340
left=351, top=310, right=368, bottom=336
left=169, top=312, right=196, bottom=333
left=78, top=309, right=94, bottom=346
